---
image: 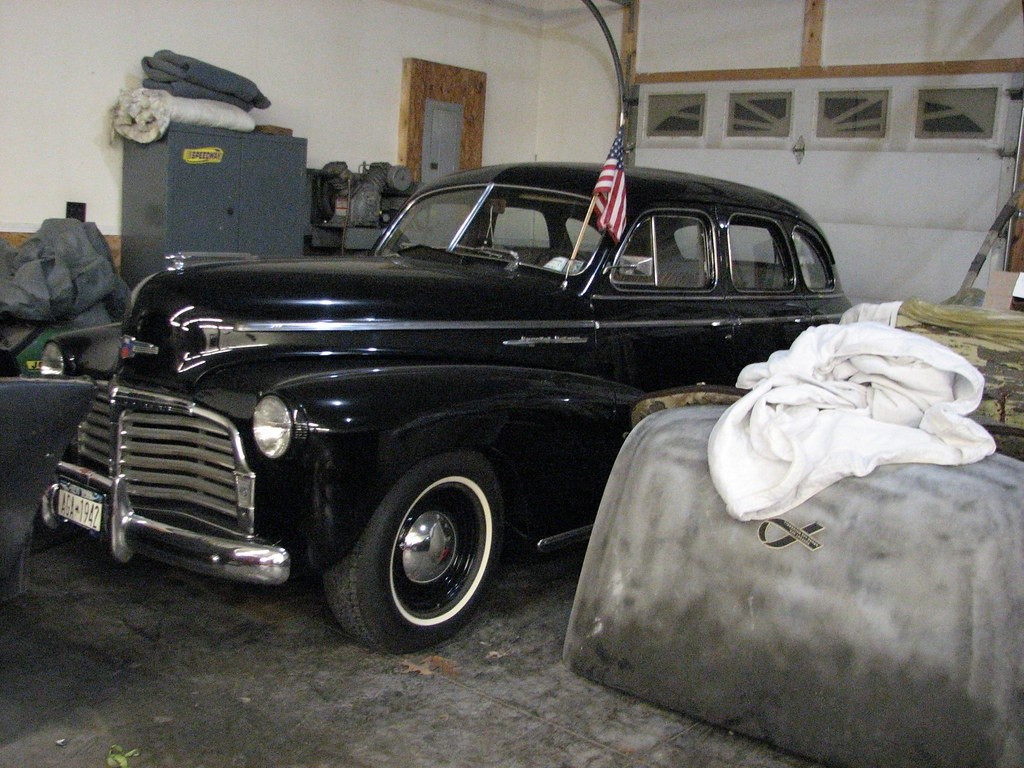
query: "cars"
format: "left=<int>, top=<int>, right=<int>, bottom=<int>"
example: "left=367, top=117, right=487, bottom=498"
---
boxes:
left=30, top=161, right=863, bottom=652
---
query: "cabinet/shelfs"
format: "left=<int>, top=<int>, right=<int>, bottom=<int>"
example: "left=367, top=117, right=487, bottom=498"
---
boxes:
left=121, top=122, right=307, bottom=291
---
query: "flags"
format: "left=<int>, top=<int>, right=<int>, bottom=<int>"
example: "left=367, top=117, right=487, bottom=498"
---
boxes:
left=591, top=123, right=628, bottom=244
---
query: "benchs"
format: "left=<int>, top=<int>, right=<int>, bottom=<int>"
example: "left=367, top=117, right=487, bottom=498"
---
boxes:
left=728, top=261, right=786, bottom=291
left=642, top=261, right=707, bottom=289
